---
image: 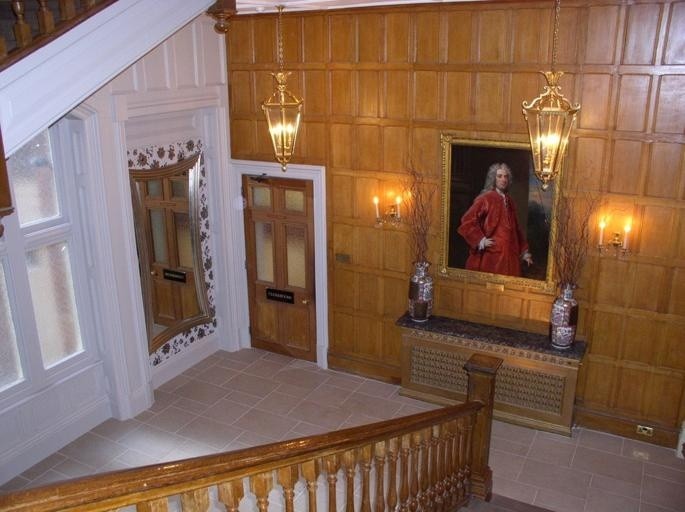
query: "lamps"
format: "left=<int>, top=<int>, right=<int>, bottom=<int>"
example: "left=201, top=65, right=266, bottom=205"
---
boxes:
left=521, top=0, right=582, bottom=190
left=262, top=6, right=307, bottom=172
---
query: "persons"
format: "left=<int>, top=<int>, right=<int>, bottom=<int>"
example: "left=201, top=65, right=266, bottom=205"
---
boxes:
left=457, top=162, right=535, bottom=277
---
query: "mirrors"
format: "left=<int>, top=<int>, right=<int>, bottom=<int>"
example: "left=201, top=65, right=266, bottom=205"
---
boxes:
left=128, top=152, right=211, bottom=353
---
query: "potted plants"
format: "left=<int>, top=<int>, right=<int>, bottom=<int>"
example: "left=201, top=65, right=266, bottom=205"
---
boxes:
left=534, top=179, right=599, bottom=350
left=395, top=157, right=438, bottom=324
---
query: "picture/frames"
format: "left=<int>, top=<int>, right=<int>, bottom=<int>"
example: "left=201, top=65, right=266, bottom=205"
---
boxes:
left=438, top=129, right=563, bottom=295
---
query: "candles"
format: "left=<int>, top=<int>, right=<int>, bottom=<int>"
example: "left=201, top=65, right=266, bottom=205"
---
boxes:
left=597, top=215, right=633, bottom=249
left=373, top=195, right=401, bottom=220
